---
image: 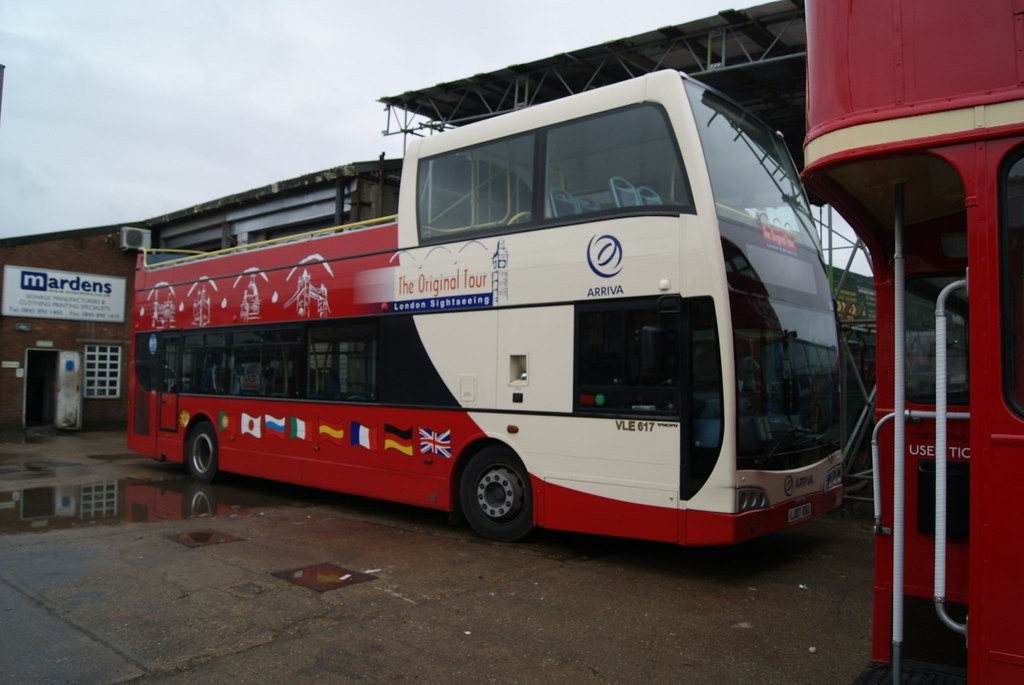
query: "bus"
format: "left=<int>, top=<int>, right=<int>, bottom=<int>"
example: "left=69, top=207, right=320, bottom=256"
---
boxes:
left=796, top=0, right=1024, bottom=685
left=125, top=69, right=848, bottom=548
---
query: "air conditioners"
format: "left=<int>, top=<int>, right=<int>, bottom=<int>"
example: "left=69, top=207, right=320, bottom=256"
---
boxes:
left=120, top=227, right=152, bottom=252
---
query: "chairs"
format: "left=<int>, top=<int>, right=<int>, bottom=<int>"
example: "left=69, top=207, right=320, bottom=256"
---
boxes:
left=209, top=364, right=334, bottom=399
left=547, top=175, right=664, bottom=220
